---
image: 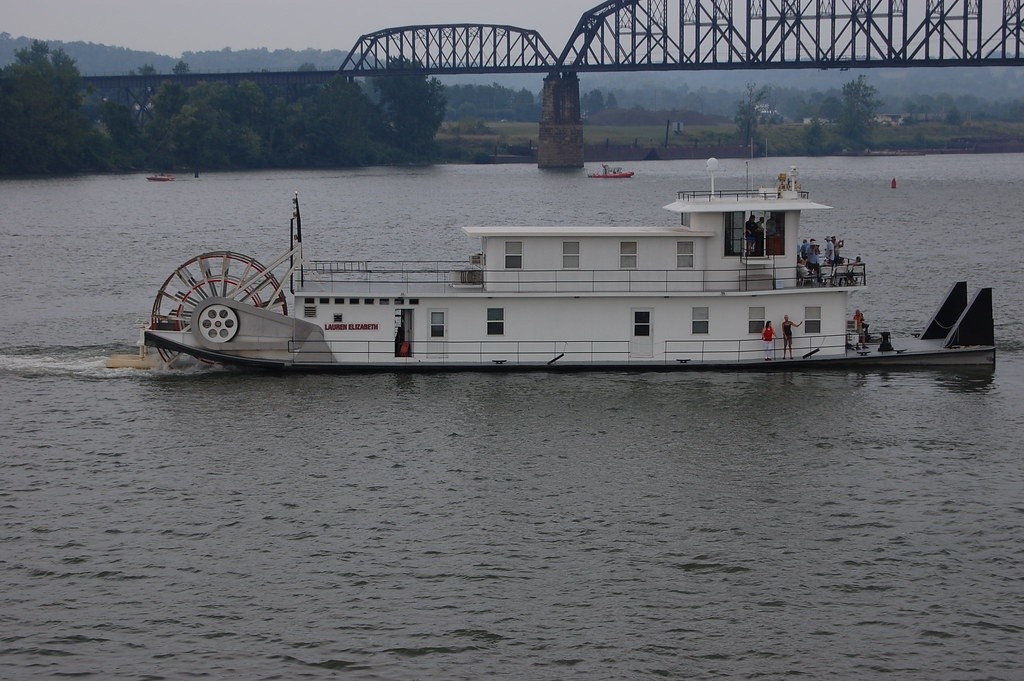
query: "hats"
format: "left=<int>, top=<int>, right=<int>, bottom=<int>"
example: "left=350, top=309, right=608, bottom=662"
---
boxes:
left=824, top=236, right=833, bottom=241
left=810, top=241, right=817, bottom=246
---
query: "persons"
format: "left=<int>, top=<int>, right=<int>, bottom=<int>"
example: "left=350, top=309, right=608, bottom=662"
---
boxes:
left=854, top=310, right=869, bottom=349
left=797, top=236, right=863, bottom=285
left=745, top=214, right=783, bottom=255
left=782, top=315, right=802, bottom=359
left=761, top=321, right=777, bottom=361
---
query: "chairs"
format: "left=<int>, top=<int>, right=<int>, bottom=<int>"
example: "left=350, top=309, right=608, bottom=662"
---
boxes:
left=797, top=266, right=819, bottom=287
left=822, top=265, right=864, bottom=286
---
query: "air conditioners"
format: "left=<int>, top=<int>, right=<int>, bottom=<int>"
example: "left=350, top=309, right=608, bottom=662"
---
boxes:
left=846, top=320, right=857, bottom=331
left=469, top=254, right=483, bottom=264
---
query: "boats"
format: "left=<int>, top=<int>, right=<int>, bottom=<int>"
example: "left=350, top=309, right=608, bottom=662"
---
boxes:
left=587, top=163, right=635, bottom=178
left=145, top=169, right=176, bottom=181
left=104, top=136, right=999, bottom=375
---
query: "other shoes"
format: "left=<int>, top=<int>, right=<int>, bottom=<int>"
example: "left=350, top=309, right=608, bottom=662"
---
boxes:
left=790, top=357, right=793, bottom=360
left=783, top=358, right=786, bottom=359
left=765, top=358, right=771, bottom=361
